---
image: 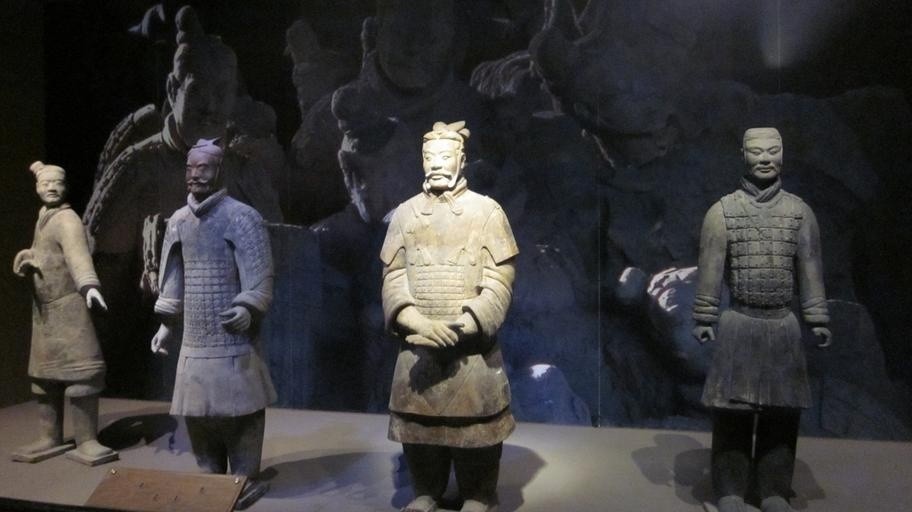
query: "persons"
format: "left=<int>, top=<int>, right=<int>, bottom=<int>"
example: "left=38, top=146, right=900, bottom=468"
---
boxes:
left=81, top=2, right=910, bottom=445
left=150, top=139, right=276, bottom=500
left=381, top=120, right=519, bottom=511
left=10, top=162, right=117, bottom=458
left=692, top=127, right=834, bottom=511
